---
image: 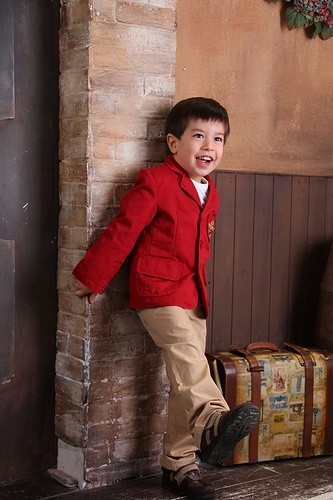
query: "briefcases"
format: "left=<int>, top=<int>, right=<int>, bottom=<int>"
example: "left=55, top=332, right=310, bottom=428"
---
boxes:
left=203, top=341, right=333, bottom=466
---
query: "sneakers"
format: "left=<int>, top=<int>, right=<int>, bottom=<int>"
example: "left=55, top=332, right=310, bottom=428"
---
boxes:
left=200, top=403, right=259, bottom=464
left=160, top=465, right=216, bottom=500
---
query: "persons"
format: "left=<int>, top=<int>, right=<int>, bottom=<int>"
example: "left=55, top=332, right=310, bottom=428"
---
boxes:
left=73, top=98, right=261, bottom=500
